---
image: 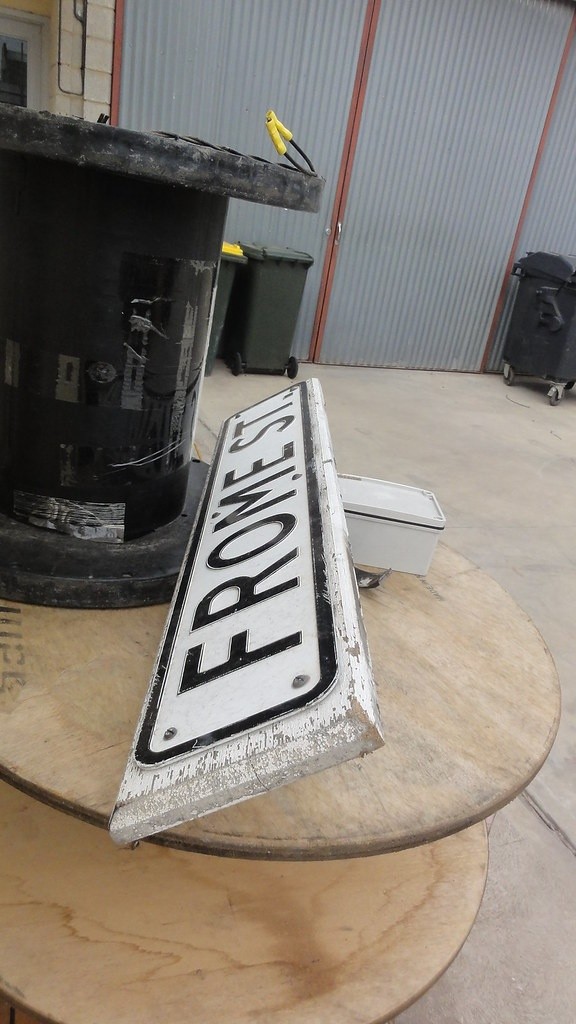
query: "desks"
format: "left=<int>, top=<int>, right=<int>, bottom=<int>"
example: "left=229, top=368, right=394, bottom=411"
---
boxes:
left=0, top=541, right=561, bottom=1024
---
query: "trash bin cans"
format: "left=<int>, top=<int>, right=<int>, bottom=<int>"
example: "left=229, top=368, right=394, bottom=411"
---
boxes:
left=500, top=247, right=576, bottom=407
left=202, top=240, right=316, bottom=381
